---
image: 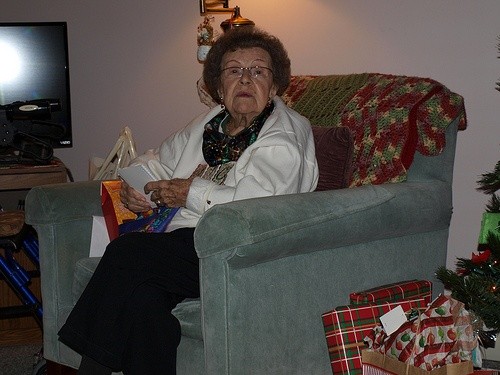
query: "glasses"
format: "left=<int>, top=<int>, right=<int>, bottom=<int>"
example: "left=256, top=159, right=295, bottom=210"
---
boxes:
left=219, top=66, right=274, bottom=79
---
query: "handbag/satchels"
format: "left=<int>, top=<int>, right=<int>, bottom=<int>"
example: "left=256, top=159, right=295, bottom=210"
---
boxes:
left=119, top=206, right=180, bottom=236
left=100, top=181, right=154, bottom=242
left=13, top=131, right=74, bottom=182
left=362, top=307, right=474, bottom=375
left=0, top=98, right=68, bottom=146
left=88, top=126, right=139, bottom=181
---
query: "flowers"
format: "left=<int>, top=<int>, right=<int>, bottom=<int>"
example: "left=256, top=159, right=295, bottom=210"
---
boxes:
left=196, top=15, right=216, bottom=45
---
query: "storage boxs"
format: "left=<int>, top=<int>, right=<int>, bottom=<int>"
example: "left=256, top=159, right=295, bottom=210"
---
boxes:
left=321, top=280, right=473, bottom=375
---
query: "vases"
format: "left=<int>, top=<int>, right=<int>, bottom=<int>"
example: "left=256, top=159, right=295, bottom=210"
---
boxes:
left=198, top=42, right=212, bottom=61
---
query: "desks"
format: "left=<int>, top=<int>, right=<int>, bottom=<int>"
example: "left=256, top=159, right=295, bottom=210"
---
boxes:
left=0, top=160, right=68, bottom=273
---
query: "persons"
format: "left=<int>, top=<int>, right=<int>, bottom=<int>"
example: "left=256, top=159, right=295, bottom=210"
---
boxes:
left=56, top=25, right=319, bottom=375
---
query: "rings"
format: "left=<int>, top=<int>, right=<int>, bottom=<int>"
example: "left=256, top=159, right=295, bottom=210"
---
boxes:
left=156, top=200, right=161, bottom=205
left=126, top=203, right=128, bottom=208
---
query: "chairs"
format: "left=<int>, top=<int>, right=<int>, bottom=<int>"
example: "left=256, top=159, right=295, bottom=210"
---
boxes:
left=0, top=209, right=42, bottom=318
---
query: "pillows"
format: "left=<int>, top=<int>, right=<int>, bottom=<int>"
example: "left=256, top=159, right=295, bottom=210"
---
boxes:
left=311, top=123, right=356, bottom=190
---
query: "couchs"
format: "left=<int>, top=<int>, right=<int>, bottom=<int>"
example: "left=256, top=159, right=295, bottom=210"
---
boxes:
left=24, top=72, right=467, bottom=375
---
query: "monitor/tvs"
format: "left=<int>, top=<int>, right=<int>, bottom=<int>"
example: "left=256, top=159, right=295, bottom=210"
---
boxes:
left=0, top=22, right=73, bottom=152
